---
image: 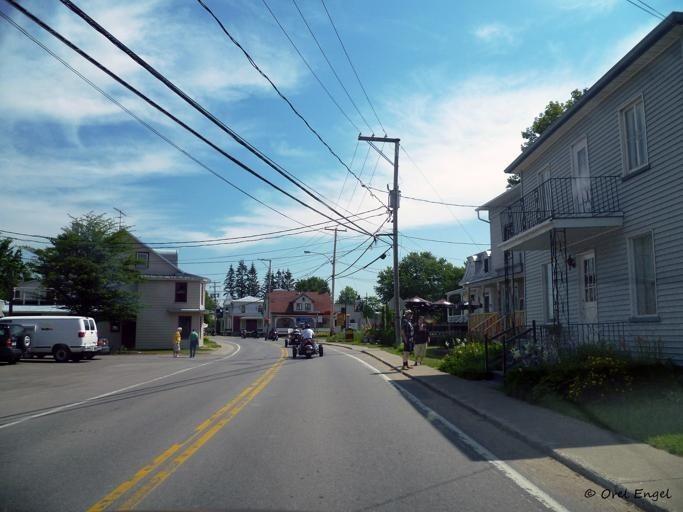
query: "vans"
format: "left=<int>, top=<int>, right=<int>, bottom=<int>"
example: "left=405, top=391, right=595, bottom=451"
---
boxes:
left=0, top=316, right=98, bottom=361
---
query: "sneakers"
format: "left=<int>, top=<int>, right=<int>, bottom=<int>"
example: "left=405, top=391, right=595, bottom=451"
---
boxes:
left=402, top=366, right=411, bottom=370
left=414, top=362, right=417, bottom=366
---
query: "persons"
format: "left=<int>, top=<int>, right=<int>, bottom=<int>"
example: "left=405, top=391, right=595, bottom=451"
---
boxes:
left=172, top=327, right=183, bottom=358
left=203, top=325, right=216, bottom=335
left=240, top=327, right=279, bottom=341
left=400, top=309, right=415, bottom=370
left=412, top=316, right=430, bottom=366
left=188, top=329, right=199, bottom=358
left=293, top=324, right=300, bottom=333
left=299, top=323, right=318, bottom=354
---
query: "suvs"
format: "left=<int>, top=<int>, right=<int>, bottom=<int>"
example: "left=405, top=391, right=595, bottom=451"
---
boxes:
left=0, top=324, right=31, bottom=365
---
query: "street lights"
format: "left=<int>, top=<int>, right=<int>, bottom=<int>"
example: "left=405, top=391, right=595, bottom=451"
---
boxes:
left=257, top=258, right=270, bottom=340
left=303, top=251, right=334, bottom=337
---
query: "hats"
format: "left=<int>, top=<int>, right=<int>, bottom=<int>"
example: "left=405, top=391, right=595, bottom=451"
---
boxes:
left=405, top=309, right=413, bottom=315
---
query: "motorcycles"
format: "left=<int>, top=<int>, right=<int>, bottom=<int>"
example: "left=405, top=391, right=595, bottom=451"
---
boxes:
left=268, top=329, right=278, bottom=341
left=245, top=330, right=256, bottom=338
left=284, top=331, right=322, bottom=359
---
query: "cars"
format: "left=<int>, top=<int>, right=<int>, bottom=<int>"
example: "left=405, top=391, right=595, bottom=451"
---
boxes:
left=95, top=337, right=109, bottom=355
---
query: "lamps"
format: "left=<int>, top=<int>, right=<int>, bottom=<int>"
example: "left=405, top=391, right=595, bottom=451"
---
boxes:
left=567, top=254, right=576, bottom=270
